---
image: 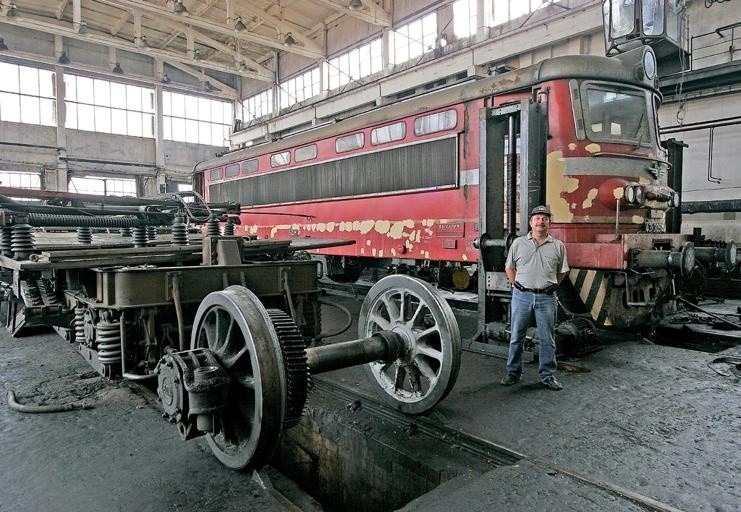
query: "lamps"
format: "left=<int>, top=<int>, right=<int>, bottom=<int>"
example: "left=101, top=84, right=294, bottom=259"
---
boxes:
left=435, top=33, right=448, bottom=48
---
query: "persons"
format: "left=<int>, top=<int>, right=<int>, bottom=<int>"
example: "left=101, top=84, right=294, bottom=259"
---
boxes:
left=500, top=204, right=571, bottom=392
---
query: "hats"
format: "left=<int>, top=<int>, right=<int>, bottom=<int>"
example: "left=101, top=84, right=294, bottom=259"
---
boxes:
left=531, top=205, right=552, bottom=217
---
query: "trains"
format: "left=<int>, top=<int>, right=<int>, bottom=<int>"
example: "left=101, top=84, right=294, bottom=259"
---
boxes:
left=189, top=41, right=738, bottom=352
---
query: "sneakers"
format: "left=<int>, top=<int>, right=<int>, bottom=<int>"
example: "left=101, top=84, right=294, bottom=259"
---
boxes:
left=541, top=376, right=564, bottom=391
left=501, top=373, right=521, bottom=386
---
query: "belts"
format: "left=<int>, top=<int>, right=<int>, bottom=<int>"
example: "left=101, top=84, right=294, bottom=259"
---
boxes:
left=514, top=281, right=559, bottom=294
